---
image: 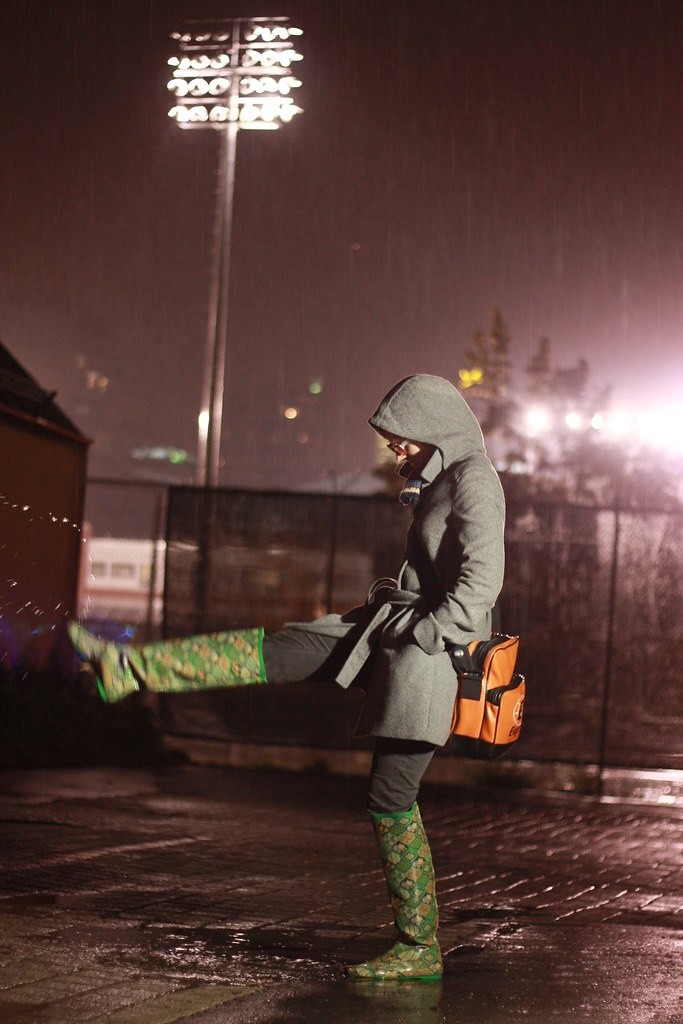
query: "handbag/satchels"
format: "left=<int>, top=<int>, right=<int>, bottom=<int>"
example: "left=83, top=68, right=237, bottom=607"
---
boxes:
left=450, top=631, right=526, bottom=761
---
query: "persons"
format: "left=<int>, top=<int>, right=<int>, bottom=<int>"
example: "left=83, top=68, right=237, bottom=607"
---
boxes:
left=65, top=368, right=506, bottom=983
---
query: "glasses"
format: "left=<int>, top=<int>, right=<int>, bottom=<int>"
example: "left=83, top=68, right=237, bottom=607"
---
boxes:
left=387, top=439, right=410, bottom=455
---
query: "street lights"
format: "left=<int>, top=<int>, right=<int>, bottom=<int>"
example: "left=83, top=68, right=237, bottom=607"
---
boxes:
left=158, top=10, right=309, bottom=641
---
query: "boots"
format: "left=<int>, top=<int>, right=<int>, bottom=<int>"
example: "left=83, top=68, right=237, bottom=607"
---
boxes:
left=66, top=620, right=271, bottom=706
left=344, top=801, right=444, bottom=979
